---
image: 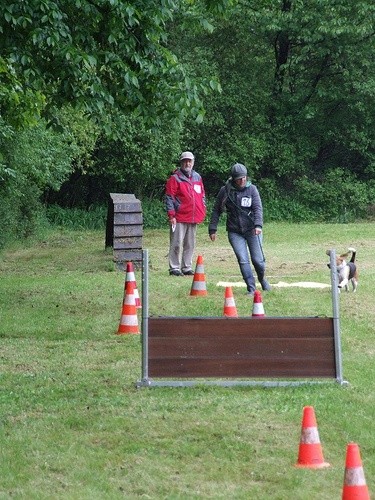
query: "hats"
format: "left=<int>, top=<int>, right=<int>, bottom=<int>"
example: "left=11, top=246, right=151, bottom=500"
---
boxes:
left=179, top=151, right=194, bottom=161
left=232, top=163, right=247, bottom=180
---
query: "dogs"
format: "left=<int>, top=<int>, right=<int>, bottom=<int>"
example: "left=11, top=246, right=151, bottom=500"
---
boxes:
left=326, top=246, right=360, bottom=294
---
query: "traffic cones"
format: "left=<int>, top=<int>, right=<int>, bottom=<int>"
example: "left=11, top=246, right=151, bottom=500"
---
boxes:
left=116, top=283, right=141, bottom=335
left=123, top=261, right=142, bottom=309
left=341, top=442, right=372, bottom=500
left=190, top=252, right=208, bottom=296
left=294, top=405, right=330, bottom=469
left=223, top=285, right=239, bottom=316
left=251, top=289, right=265, bottom=317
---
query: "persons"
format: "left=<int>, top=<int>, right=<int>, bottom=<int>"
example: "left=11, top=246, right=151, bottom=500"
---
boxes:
left=208, top=163, right=271, bottom=294
left=165, top=151, right=207, bottom=276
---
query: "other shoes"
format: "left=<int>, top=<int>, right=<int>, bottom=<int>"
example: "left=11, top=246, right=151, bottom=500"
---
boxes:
left=261, top=280, right=271, bottom=291
left=170, top=268, right=182, bottom=276
left=247, top=285, right=254, bottom=294
left=182, top=267, right=195, bottom=275
